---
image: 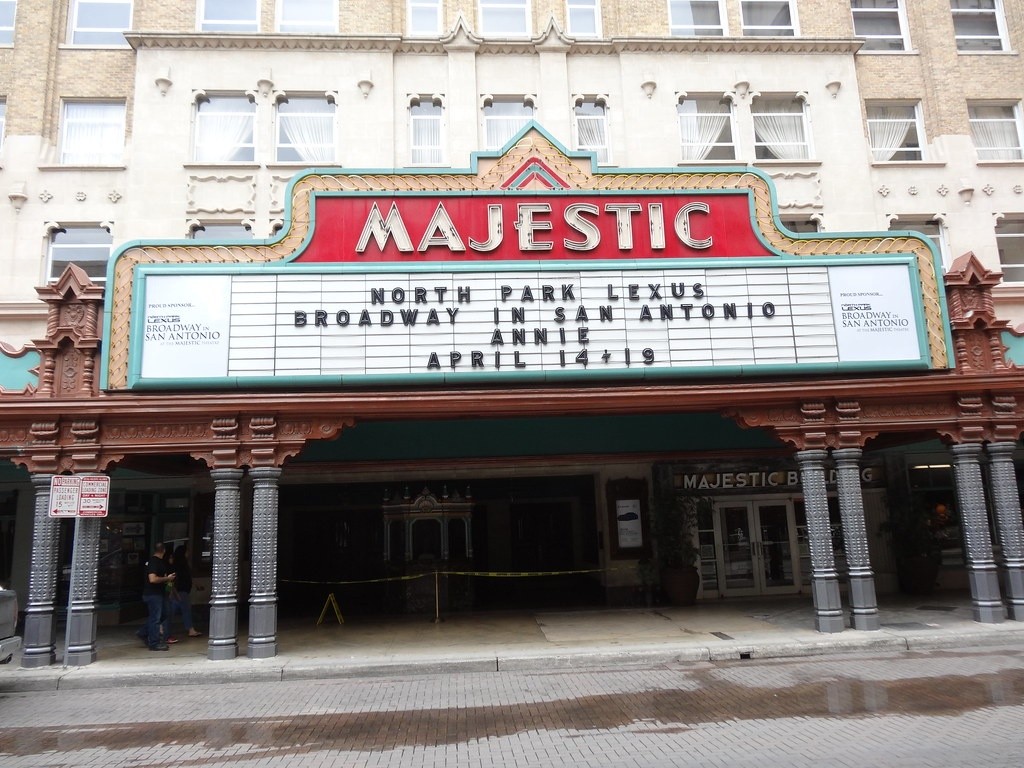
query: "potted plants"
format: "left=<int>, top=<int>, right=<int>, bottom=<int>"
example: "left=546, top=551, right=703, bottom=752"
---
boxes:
left=876, top=483, right=949, bottom=594
left=629, top=559, right=665, bottom=607
left=645, top=461, right=716, bottom=607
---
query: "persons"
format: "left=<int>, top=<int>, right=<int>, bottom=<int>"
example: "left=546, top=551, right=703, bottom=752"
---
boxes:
left=160, top=552, right=177, bottom=643
left=166, top=545, right=202, bottom=637
left=137, top=542, right=168, bottom=650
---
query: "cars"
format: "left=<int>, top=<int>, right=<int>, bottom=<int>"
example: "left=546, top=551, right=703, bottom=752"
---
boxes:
left=0, top=582, right=22, bottom=665
left=61, top=546, right=156, bottom=598
left=153, top=536, right=248, bottom=606
left=618, top=512, right=638, bottom=521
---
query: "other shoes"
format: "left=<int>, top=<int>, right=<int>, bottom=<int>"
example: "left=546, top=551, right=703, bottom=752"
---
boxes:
left=149, top=642, right=168, bottom=651
left=188, top=631, right=203, bottom=637
left=159, top=635, right=178, bottom=643
left=134, top=629, right=150, bottom=646
